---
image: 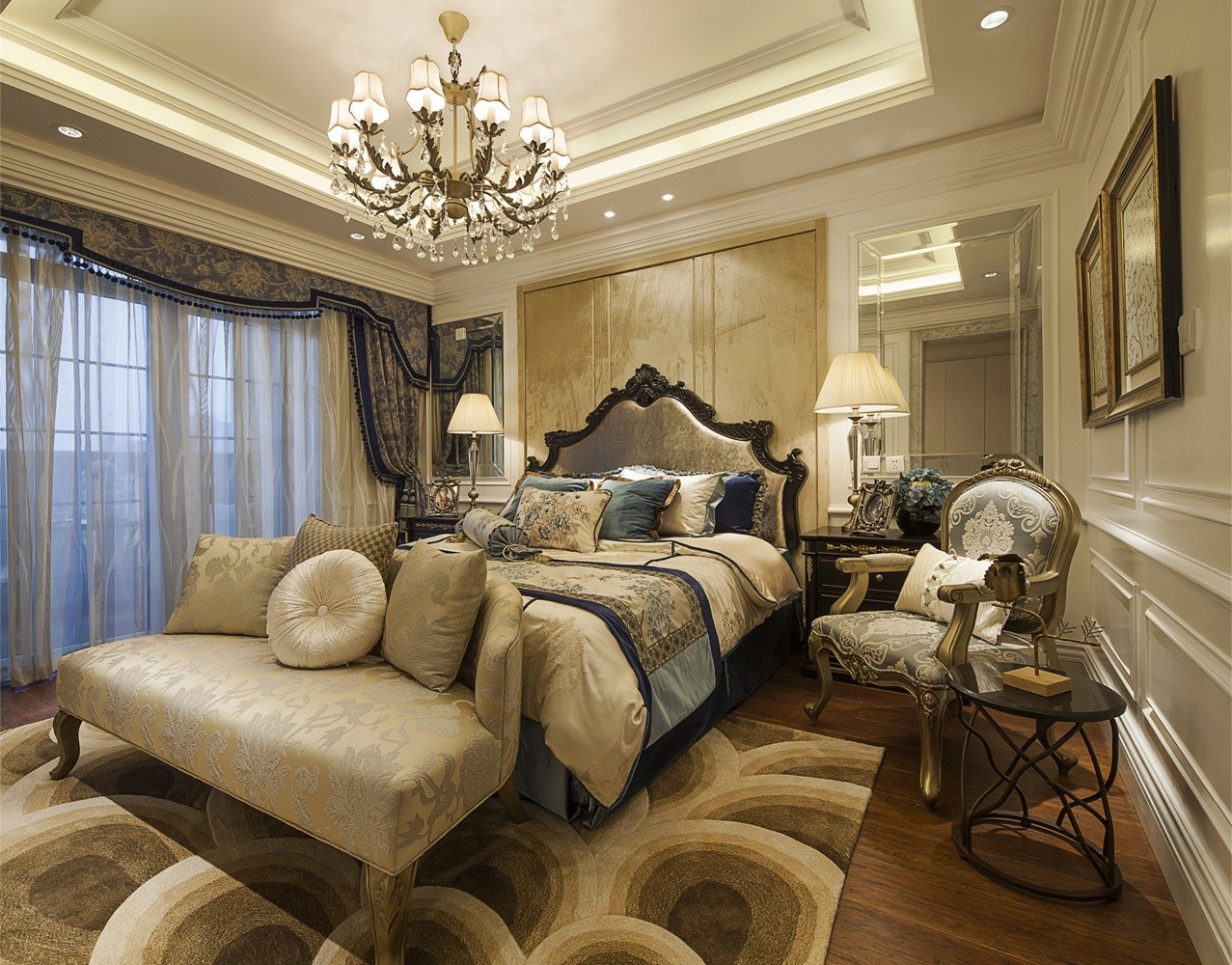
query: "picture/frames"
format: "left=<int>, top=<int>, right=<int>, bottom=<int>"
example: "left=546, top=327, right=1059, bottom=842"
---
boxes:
left=426, top=474, right=462, bottom=518
left=1098, top=74, right=1184, bottom=420
left=1074, top=192, right=1116, bottom=429
left=848, top=477, right=900, bottom=538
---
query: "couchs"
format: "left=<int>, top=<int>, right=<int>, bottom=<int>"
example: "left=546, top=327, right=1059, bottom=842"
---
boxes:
left=49, top=548, right=525, bottom=965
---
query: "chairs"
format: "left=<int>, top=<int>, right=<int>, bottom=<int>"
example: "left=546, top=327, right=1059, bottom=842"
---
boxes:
left=803, top=459, right=1085, bottom=810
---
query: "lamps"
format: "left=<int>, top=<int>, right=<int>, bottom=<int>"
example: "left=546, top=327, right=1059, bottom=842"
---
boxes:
left=447, top=392, right=504, bottom=517
left=859, top=367, right=914, bottom=479
left=325, top=10, right=573, bottom=267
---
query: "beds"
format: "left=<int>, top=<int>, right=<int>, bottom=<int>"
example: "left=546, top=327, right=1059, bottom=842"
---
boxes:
left=396, top=363, right=811, bottom=830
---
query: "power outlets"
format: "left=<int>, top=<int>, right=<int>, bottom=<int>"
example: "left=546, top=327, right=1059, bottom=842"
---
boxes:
left=813, top=351, right=900, bottom=533
left=885, top=456, right=905, bottom=473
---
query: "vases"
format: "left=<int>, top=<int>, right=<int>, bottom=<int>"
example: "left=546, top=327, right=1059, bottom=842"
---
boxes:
left=894, top=503, right=942, bottom=535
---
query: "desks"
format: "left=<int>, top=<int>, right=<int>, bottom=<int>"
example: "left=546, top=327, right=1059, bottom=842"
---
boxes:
left=942, top=663, right=1129, bottom=905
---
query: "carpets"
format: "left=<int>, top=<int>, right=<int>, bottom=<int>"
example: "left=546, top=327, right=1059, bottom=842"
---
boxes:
left=1, top=715, right=888, bottom=965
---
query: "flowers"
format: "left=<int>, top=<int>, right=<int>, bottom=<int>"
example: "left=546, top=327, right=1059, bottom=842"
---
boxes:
left=889, top=466, right=955, bottom=517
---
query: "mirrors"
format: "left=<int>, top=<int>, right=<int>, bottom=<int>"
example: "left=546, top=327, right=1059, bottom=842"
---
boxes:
left=856, top=198, right=1046, bottom=482
left=430, top=301, right=512, bottom=487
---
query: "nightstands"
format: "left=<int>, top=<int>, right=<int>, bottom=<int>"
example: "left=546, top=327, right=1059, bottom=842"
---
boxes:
left=399, top=513, right=466, bottom=544
left=797, top=525, right=946, bottom=693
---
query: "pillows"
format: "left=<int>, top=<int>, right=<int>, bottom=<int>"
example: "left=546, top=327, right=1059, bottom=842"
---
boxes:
left=161, top=533, right=298, bottom=639
left=618, top=467, right=730, bottom=537
left=500, top=470, right=596, bottom=523
left=282, top=511, right=399, bottom=585
left=516, top=486, right=613, bottom=554
left=496, top=467, right=622, bottom=522
left=266, top=549, right=388, bottom=669
left=379, top=537, right=488, bottom=693
left=446, top=507, right=543, bottom=561
left=631, top=464, right=768, bottom=539
left=895, top=542, right=1013, bottom=647
